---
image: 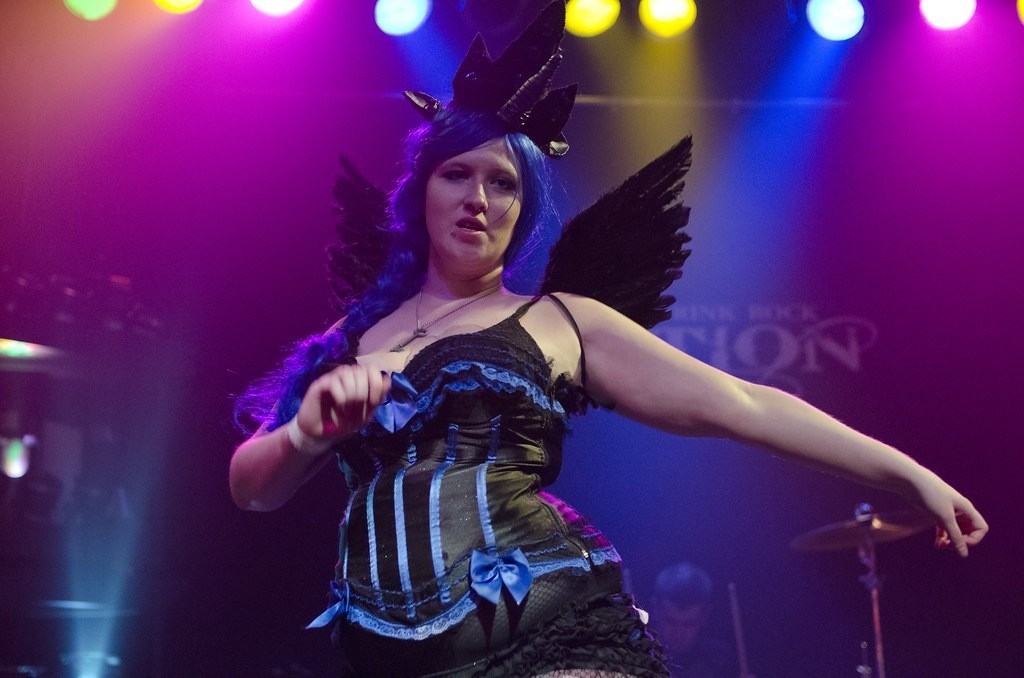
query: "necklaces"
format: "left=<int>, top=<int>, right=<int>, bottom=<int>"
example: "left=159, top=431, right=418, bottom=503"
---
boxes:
left=390, top=275, right=503, bottom=353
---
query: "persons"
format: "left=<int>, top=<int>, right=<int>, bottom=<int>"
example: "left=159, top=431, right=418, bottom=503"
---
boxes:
left=229, top=106, right=988, bottom=678
left=650, top=564, right=755, bottom=678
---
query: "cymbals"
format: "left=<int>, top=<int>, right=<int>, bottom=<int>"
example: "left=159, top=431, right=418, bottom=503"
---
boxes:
left=784, top=514, right=954, bottom=556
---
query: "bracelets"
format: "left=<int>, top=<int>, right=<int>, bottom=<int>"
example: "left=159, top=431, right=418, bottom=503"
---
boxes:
left=288, top=414, right=332, bottom=455
